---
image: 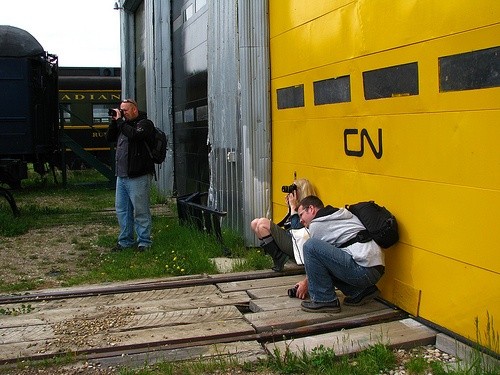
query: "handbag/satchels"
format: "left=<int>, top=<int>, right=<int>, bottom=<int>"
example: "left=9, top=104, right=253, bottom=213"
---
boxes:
left=289, top=226, right=311, bottom=265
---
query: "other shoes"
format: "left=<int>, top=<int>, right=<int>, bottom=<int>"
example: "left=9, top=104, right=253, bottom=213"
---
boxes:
left=112, top=244, right=128, bottom=252
left=137, top=245, right=147, bottom=253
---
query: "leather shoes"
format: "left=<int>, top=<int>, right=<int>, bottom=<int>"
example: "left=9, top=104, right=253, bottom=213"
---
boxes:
left=301, top=297, right=341, bottom=314
left=343, top=283, right=381, bottom=306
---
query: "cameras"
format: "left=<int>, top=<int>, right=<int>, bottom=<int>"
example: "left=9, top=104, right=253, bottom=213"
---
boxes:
left=282, top=184, right=296, bottom=194
left=109, top=109, right=124, bottom=118
left=288, top=286, right=298, bottom=298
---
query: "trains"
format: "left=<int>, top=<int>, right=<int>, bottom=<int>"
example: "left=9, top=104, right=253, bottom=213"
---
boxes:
left=0, top=25, right=122, bottom=161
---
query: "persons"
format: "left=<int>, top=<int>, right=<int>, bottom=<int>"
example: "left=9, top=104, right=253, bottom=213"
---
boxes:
left=105, top=98, right=154, bottom=253
left=248, top=179, right=316, bottom=271
left=293, top=195, right=385, bottom=314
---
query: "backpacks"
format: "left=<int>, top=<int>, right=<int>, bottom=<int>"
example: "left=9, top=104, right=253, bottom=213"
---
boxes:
left=136, top=118, right=168, bottom=182
left=335, top=200, right=400, bottom=250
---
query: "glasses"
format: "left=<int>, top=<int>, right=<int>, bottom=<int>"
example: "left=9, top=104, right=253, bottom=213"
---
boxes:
left=298, top=207, right=307, bottom=217
left=119, top=99, right=137, bottom=106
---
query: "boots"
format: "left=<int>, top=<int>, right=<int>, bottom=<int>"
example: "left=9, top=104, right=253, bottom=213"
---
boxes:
left=260, top=240, right=290, bottom=273
